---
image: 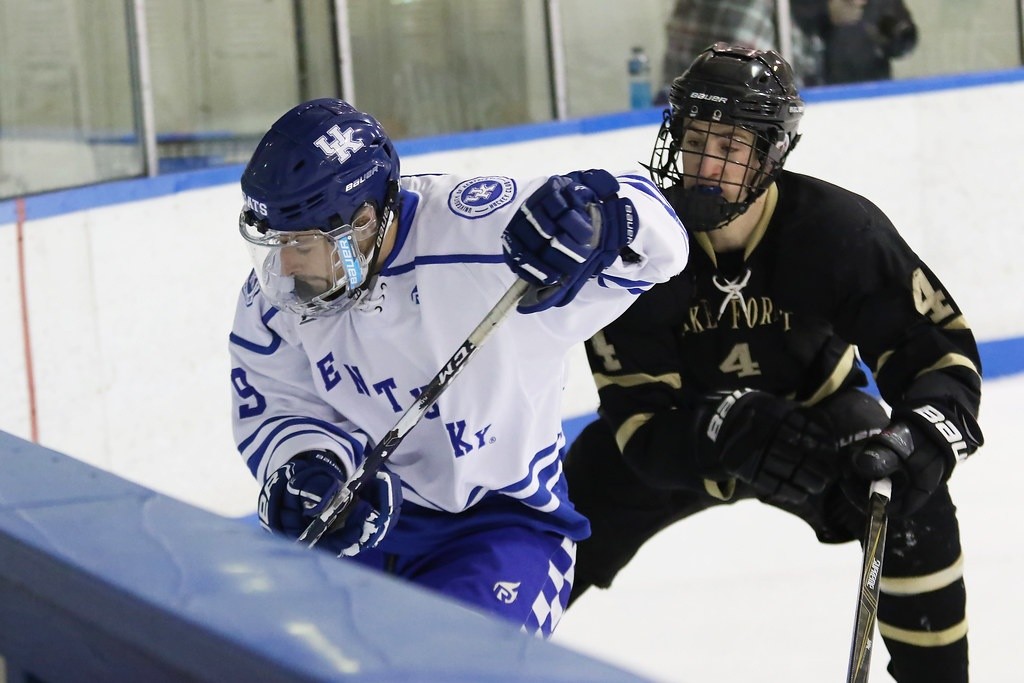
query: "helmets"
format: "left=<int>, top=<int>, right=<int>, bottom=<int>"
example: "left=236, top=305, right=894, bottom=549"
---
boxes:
left=239, top=97, right=400, bottom=318
left=642, top=42, right=806, bottom=234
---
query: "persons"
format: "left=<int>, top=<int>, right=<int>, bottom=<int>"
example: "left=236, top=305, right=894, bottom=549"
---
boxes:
left=563, top=42, right=984, bottom=683
left=655, top=0, right=918, bottom=106
left=229, top=98, right=689, bottom=642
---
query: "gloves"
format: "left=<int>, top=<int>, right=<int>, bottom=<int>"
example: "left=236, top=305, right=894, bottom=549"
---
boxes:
left=843, top=396, right=985, bottom=534
left=500, top=169, right=639, bottom=314
left=703, top=389, right=852, bottom=506
left=257, top=449, right=401, bottom=559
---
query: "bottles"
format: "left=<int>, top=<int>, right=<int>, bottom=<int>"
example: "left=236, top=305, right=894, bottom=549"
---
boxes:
left=628, top=46, right=652, bottom=110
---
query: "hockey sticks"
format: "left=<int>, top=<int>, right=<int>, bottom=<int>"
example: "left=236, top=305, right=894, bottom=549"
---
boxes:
left=293, top=202, right=605, bottom=550
left=845, top=477, right=898, bottom=683
